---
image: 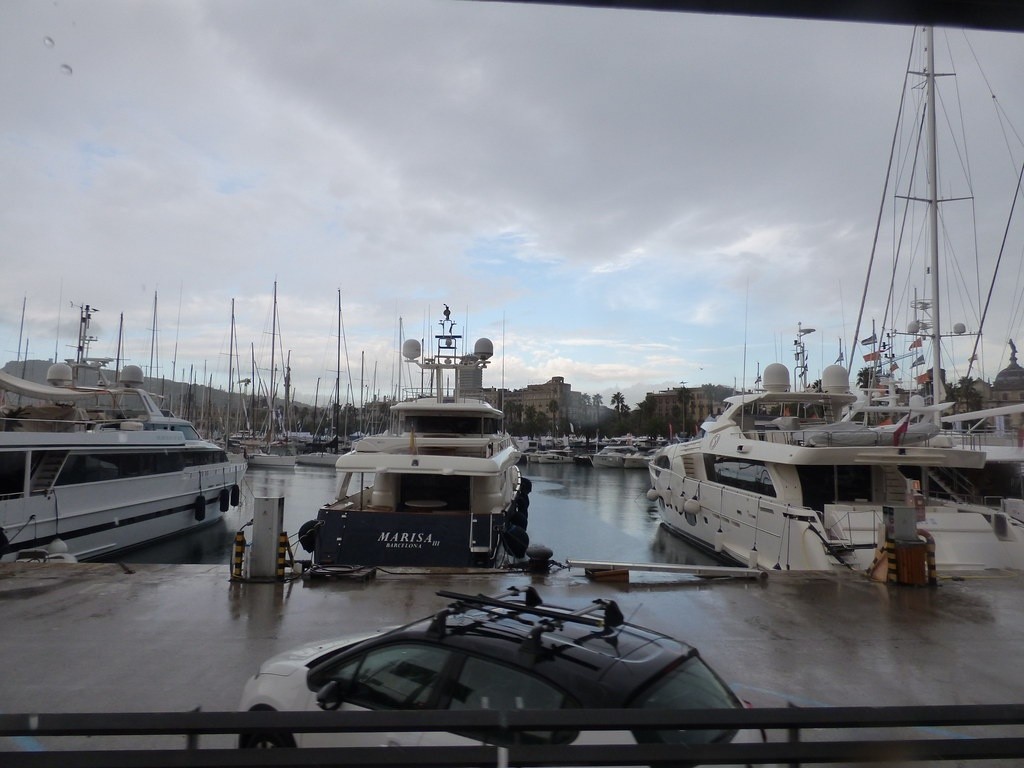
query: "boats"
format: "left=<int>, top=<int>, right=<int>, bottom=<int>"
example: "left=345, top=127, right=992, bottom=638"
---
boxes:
left=0, top=284, right=249, bottom=564
left=299, top=297, right=533, bottom=567
left=511, top=432, right=687, bottom=468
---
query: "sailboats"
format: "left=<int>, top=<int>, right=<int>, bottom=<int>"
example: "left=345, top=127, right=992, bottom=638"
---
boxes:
left=644, top=26, right=1024, bottom=570
left=142, top=290, right=410, bottom=469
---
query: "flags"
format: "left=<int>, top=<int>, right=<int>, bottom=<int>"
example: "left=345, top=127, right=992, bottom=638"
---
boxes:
left=893, top=409, right=911, bottom=446
left=891, top=362, right=899, bottom=372
left=914, top=371, right=930, bottom=384
left=863, top=352, right=879, bottom=362
left=909, top=337, right=922, bottom=351
left=862, top=334, right=877, bottom=345
left=755, top=376, right=761, bottom=384
left=834, top=352, right=844, bottom=364
left=911, top=355, right=925, bottom=368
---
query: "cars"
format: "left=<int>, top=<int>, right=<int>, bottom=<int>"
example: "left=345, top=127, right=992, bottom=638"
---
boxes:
left=235, top=584, right=773, bottom=768
